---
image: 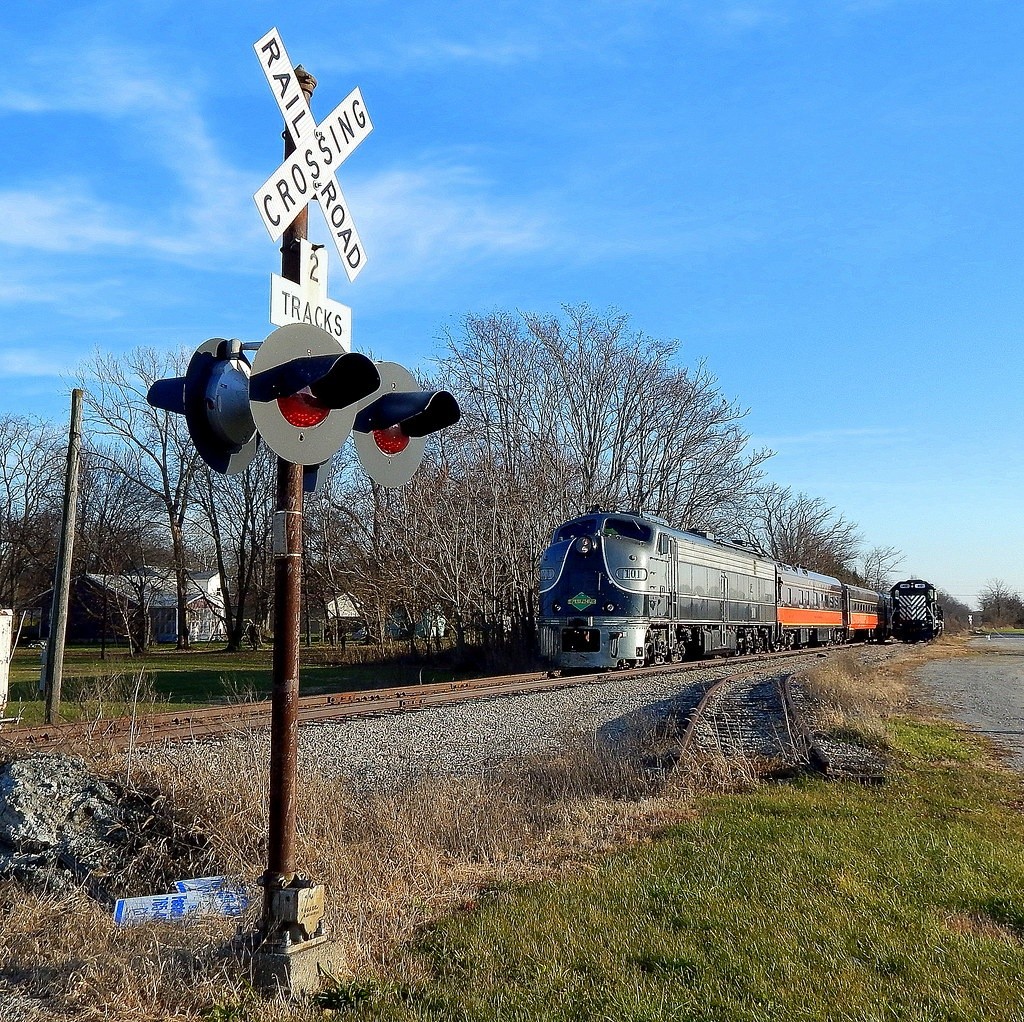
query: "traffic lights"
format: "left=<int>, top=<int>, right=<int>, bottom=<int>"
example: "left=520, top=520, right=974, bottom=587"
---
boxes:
left=144, top=337, right=460, bottom=488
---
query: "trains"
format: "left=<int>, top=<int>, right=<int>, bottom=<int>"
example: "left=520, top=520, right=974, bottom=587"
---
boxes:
left=533, top=505, right=895, bottom=672
left=889, top=579, right=945, bottom=645
